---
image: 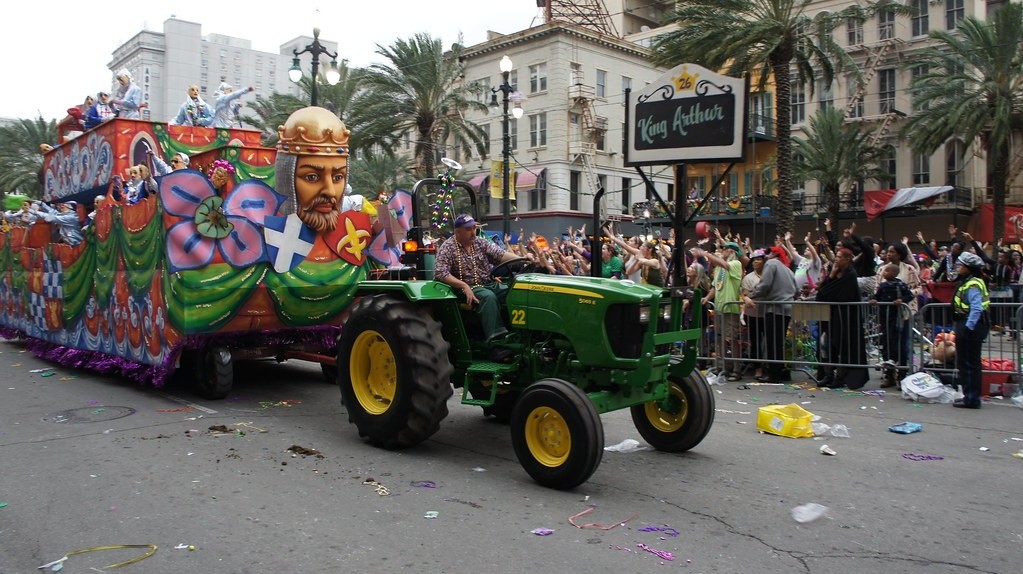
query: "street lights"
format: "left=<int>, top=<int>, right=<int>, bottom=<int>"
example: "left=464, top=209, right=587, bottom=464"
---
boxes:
left=488, top=54, right=526, bottom=242
left=288, top=26, right=341, bottom=106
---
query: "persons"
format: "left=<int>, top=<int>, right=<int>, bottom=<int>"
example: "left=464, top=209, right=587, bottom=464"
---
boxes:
left=81, top=195, right=106, bottom=231
left=175, top=85, right=216, bottom=128
left=82, top=90, right=115, bottom=130
left=108, top=68, right=142, bottom=119
left=503, top=224, right=643, bottom=285
left=0, top=199, right=84, bottom=247
left=916, top=224, right=1023, bottom=341
left=274, top=107, right=353, bottom=230
left=433, top=214, right=533, bottom=365
left=120, top=141, right=191, bottom=205
left=602, top=225, right=926, bottom=391
left=212, top=82, right=254, bottom=128
left=953, top=251, right=994, bottom=409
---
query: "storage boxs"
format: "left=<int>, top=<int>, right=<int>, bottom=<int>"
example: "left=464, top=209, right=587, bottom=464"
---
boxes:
left=982, top=372, right=1010, bottom=398
left=757, top=403, right=816, bottom=439
left=760, top=207, right=771, bottom=217
left=1002, top=383, right=1020, bottom=397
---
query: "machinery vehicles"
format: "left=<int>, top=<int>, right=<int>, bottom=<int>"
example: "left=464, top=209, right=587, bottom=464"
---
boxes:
left=330, top=173, right=717, bottom=492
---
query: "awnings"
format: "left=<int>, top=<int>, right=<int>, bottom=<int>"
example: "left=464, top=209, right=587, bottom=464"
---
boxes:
left=467, top=175, right=487, bottom=193
left=487, top=171, right=516, bottom=195
left=515, top=167, right=546, bottom=192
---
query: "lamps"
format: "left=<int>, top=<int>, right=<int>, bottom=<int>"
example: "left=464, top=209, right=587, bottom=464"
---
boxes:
left=397, top=240, right=419, bottom=265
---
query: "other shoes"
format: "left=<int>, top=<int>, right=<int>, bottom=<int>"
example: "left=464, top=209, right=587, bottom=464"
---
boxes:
left=817, top=376, right=833, bottom=386
left=880, top=378, right=904, bottom=392
left=826, top=381, right=842, bottom=387
left=486, top=348, right=514, bottom=362
left=758, top=376, right=783, bottom=383
left=728, top=372, right=742, bottom=381
left=953, top=398, right=980, bottom=408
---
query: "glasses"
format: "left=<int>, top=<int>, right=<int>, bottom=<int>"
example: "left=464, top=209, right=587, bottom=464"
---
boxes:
left=100, top=94, right=108, bottom=97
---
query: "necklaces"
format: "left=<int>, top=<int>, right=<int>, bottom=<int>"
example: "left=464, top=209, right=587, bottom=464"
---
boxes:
left=454, top=235, right=484, bottom=293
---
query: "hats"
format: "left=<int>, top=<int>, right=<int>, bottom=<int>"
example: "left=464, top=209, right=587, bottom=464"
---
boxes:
left=958, top=252, right=985, bottom=269
left=723, top=242, right=742, bottom=256
left=749, top=249, right=765, bottom=258
left=455, top=214, right=480, bottom=228
